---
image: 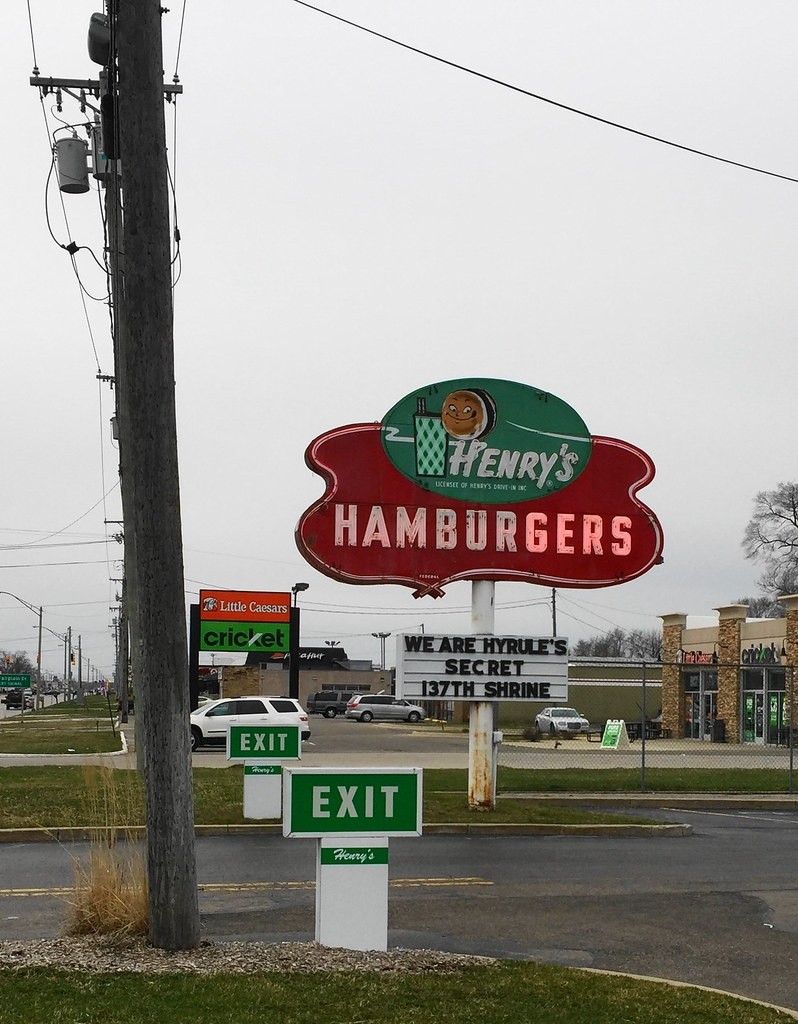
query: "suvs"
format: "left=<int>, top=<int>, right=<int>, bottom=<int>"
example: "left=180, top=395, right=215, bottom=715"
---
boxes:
left=306, top=688, right=375, bottom=718
left=190, top=696, right=312, bottom=752
left=344, top=694, right=426, bottom=723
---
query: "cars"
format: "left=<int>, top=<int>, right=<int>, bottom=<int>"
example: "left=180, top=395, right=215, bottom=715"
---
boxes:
left=5, top=688, right=35, bottom=710
left=534, top=706, right=590, bottom=736
left=198, top=695, right=224, bottom=709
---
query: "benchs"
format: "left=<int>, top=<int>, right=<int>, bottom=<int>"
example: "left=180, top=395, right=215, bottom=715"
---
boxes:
left=584, top=728, right=670, bottom=741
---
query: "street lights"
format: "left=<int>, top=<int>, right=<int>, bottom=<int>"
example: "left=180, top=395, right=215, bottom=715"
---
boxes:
left=58, top=634, right=105, bottom=696
left=32, top=625, right=72, bottom=702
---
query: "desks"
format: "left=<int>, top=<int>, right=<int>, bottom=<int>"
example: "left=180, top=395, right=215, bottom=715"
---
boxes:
left=600, top=722, right=663, bottom=741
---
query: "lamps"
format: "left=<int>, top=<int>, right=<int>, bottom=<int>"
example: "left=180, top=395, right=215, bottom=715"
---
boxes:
left=711, top=643, right=728, bottom=667
left=654, top=645, right=668, bottom=663
left=779, top=637, right=792, bottom=665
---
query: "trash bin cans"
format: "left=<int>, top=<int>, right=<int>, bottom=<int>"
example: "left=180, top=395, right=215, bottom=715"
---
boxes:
left=709, top=719, right=725, bottom=743
left=128, top=700, right=134, bottom=715
